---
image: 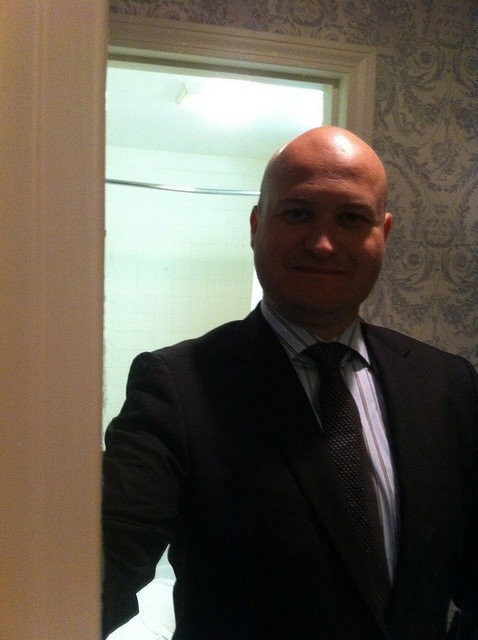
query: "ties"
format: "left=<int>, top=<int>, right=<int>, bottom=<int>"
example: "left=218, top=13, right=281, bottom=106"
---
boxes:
left=311, top=343, right=391, bottom=590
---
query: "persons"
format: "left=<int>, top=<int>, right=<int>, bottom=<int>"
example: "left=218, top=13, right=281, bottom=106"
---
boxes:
left=101, top=126, right=477, bottom=640
